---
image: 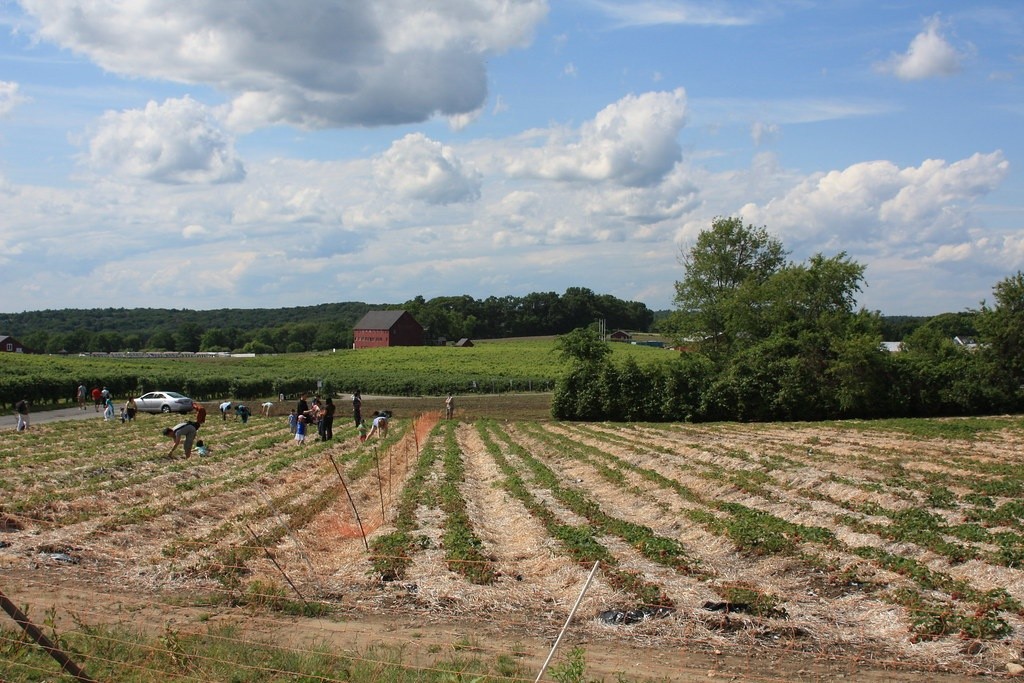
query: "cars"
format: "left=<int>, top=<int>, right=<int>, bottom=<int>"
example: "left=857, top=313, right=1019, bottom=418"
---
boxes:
left=126, top=391, right=194, bottom=415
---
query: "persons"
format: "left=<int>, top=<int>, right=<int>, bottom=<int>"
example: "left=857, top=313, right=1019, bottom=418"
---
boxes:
left=357, top=417, right=388, bottom=443
left=261, top=402, right=273, bottom=418
left=192, top=401, right=206, bottom=424
left=219, top=401, right=231, bottom=421
left=120, top=397, right=137, bottom=424
left=162, top=422, right=213, bottom=460
left=77, top=382, right=86, bottom=410
left=445, top=392, right=454, bottom=421
left=234, top=404, right=251, bottom=423
left=92, top=386, right=115, bottom=422
left=352, top=390, right=362, bottom=428
left=15, top=400, right=29, bottom=432
left=288, top=393, right=335, bottom=447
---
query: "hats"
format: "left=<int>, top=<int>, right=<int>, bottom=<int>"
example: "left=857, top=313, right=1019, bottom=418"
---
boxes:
left=298, top=415, right=306, bottom=419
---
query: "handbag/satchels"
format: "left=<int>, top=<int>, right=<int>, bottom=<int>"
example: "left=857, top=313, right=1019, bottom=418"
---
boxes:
left=187, top=421, right=200, bottom=430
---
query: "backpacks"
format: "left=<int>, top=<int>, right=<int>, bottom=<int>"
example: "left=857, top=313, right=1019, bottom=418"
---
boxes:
left=16, top=400, right=27, bottom=414
